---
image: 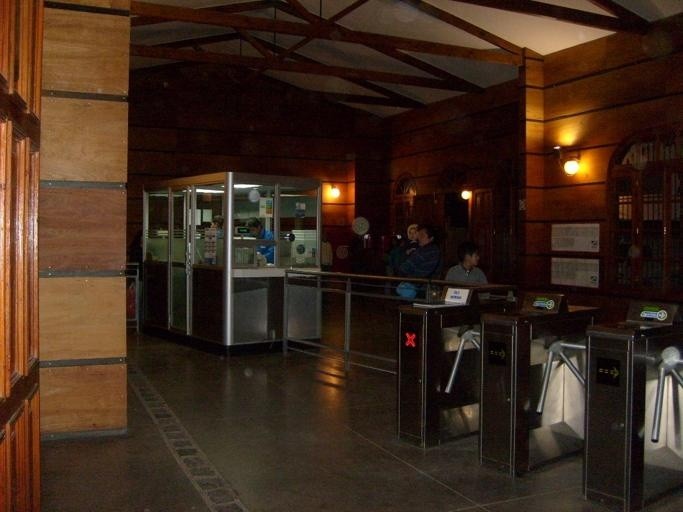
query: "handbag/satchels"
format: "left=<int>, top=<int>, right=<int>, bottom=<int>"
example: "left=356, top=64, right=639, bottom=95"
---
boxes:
left=397, top=281, right=417, bottom=299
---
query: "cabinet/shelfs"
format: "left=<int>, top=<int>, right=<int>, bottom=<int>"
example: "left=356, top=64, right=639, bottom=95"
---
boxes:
left=605, top=127, right=683, bottom=292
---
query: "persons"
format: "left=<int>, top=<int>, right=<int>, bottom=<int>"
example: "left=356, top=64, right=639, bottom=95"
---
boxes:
left=396, top=222, right=442, bottom=299
left=442, top=241, right=489, bottom=299
left=402, top=223, right=420, bottom=254
left=244, top=215, right=275, bottom=264
left=203, top=214, right=224, bottom=265
